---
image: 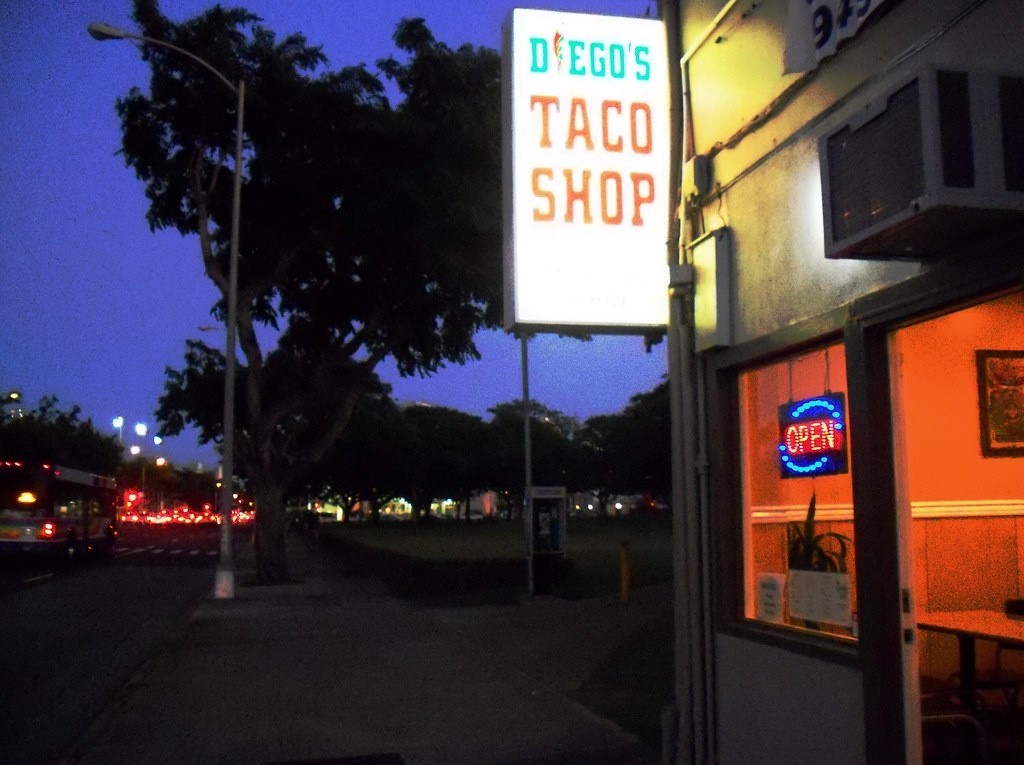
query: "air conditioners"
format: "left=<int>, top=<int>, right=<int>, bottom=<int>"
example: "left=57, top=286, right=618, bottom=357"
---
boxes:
left=812, top=60, right=1024, bottom=260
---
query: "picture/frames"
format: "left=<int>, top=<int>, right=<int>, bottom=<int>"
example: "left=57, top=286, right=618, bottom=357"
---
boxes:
left=975, top=349, right=1024, bottom=459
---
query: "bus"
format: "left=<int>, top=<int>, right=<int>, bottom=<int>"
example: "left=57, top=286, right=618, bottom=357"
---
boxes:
left=0, top=459, right=116, bottom=596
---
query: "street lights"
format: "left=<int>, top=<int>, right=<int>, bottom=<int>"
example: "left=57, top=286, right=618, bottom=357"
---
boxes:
left=88, top=21, right=248, bottom=597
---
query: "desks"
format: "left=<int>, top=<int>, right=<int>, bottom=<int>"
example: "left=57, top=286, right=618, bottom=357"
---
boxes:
left=918, top=610, right=1024, bottom=765
